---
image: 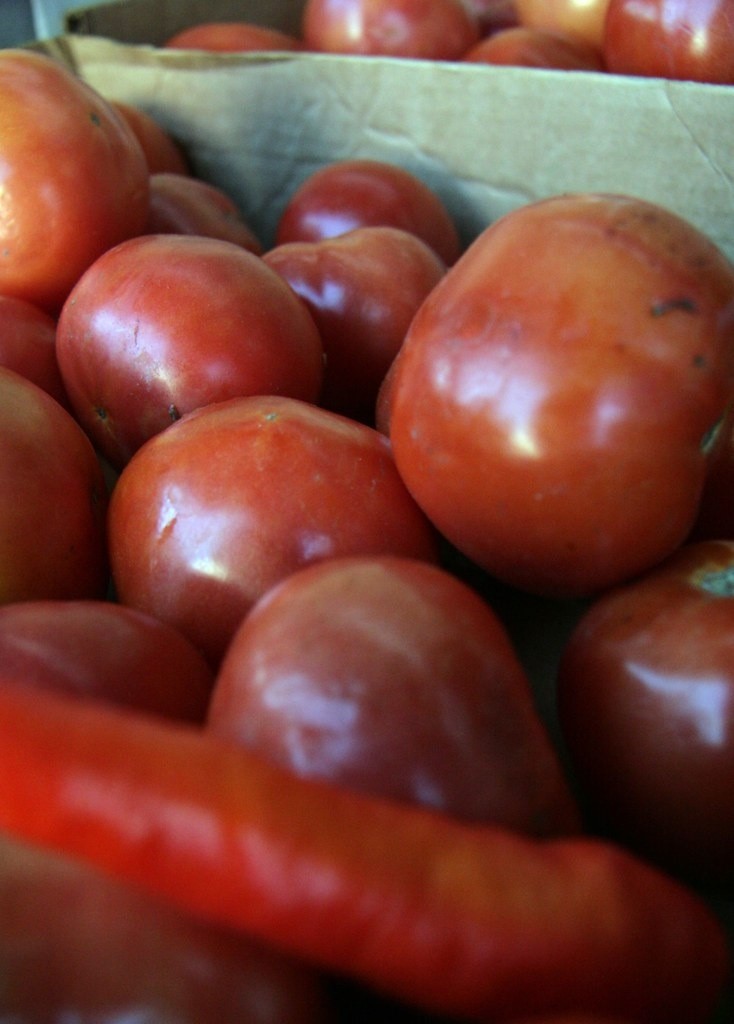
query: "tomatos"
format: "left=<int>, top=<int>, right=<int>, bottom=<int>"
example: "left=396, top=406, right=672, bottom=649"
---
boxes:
left=0, top=0, right=734, bottom=889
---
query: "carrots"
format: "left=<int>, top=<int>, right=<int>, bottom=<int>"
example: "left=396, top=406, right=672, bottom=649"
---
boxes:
left=1, top=684, right=734, bottom=1024
